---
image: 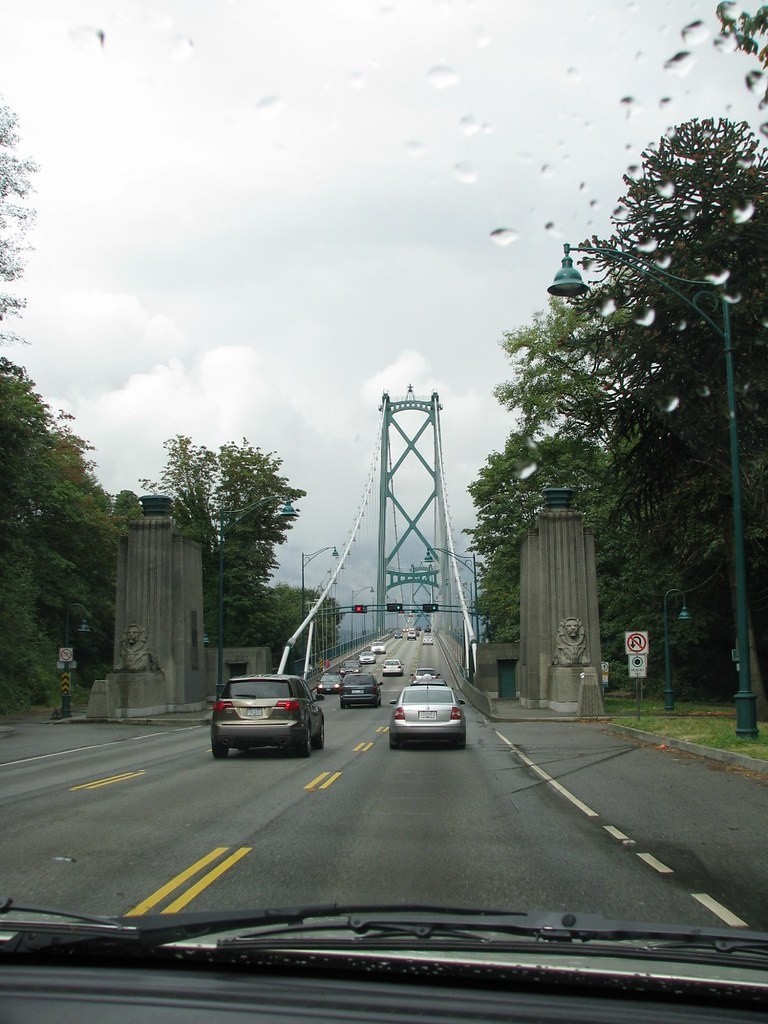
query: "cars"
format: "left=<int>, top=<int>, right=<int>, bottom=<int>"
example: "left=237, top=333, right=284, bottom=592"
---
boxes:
left=210, top=674, right=326, bottom=759
left=388, top=687, right=468, bottom=750
left=381, top=660, right=405, bottom=677
left=393, top=624, right=432, bottom=641
left=422, top=636, right=434, bottom=645
left=336, top=661, right=364, bottom=678
left=357, top=652, right=376, bottom=664
left=369, top=641, right=387, bottom=655
left=339, top=674, right=383, bottom=709
left=315, top=672, right=345, bottom=695
left=411, top=666, right=449, bottom=688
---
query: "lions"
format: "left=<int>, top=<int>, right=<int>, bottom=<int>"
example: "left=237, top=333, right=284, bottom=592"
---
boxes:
left=552, top=616, right=591, bottom=666
left=112, top=623, right=160, bottom=672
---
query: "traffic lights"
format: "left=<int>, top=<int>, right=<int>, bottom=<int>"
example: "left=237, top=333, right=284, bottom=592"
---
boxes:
left=355, top=605, right=363, bottom=613
left=387, top=603, right=402, bottom=612
left=423, top=604, right=439, bottom=612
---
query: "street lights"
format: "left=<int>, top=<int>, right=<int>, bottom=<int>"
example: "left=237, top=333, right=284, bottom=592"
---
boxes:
left=301, top=546, right=340, bottom=647
left=663, top=590, right=692, bottom=710
left=351, top=585, right=375, bottom=639
left=215, top=493, right=299, bottom=699
left=423, top=548, right=479, bottom=642
left=545, top=243, right=761, bottom=741
